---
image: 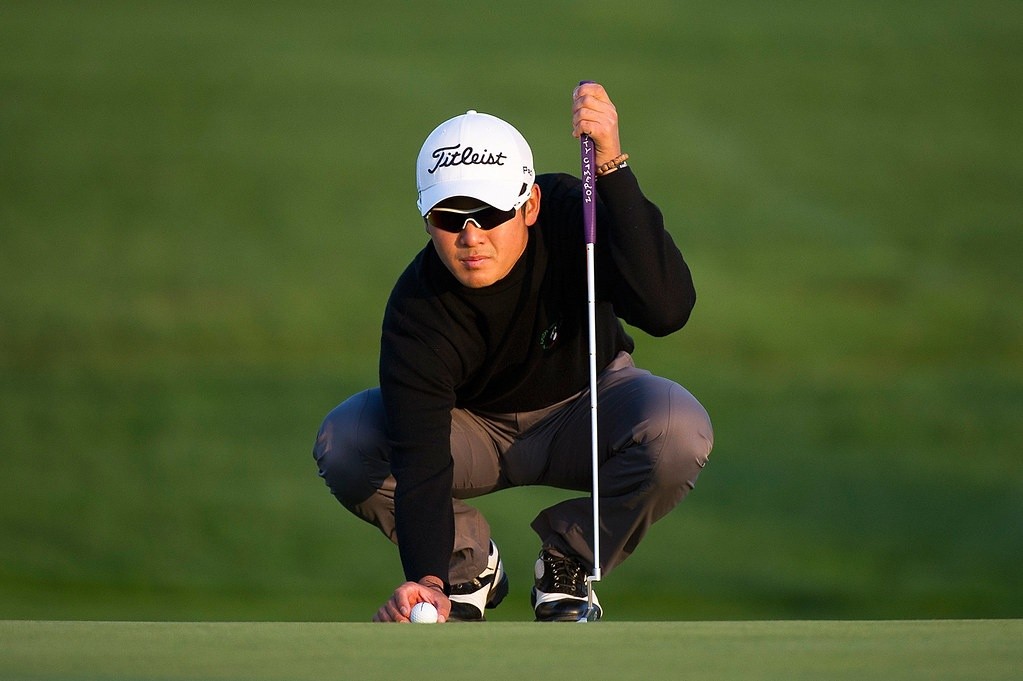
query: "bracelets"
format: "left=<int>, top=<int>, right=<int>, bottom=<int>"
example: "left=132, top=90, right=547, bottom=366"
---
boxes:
left=425, top=580, right=444, bottom=593
left=596, top=153, right=629, bottom=174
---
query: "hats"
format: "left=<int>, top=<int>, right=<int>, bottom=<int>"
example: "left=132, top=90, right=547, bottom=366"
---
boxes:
left=416, top=110, right=535, bottom=217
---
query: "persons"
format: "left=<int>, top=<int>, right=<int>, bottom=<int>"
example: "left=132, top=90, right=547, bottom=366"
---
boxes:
left=311, top=83, right=713, bottom=623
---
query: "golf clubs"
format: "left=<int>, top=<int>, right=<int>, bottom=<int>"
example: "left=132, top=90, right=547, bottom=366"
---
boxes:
left=578, top=81, right=601, bottom=625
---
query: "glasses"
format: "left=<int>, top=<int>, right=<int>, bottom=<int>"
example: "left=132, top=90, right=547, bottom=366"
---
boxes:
left=415, top=187, right=532, bottom=232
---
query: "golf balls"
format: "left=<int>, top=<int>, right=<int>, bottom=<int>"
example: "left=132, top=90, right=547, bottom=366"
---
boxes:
left=410, top=601, right=439, bottom=625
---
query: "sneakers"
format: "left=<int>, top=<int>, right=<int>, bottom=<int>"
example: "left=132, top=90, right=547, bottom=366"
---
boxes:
left=531, top=546, right=603, bottom=622
left=445, top=536, right=508, bottom=622
